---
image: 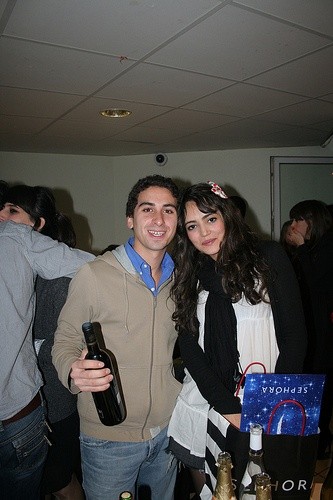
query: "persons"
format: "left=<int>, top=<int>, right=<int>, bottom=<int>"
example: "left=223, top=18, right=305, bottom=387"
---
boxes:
left=50, top=173, right=180, bottom=500
left=164, top=181, right=303, bottom=500
left=281, top=200, right=333, bottom=280
left=0, top=181, right=118, bottom=500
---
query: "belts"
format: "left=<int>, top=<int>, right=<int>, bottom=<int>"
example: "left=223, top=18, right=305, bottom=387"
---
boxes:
left=0, top=391, right=41, bottom=427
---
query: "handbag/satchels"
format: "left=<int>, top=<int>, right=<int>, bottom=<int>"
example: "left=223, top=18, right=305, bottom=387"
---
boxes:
left=226, top=362, right=325, bottom=500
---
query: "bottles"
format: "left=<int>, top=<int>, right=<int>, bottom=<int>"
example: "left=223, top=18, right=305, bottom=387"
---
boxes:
left=81, top=321, right=128, bottom=426
left=211, top=451, right=237, bottom=500
left=254, top=472, right=272, bottom=500
left=239, top=423, right=265, bottom=500
